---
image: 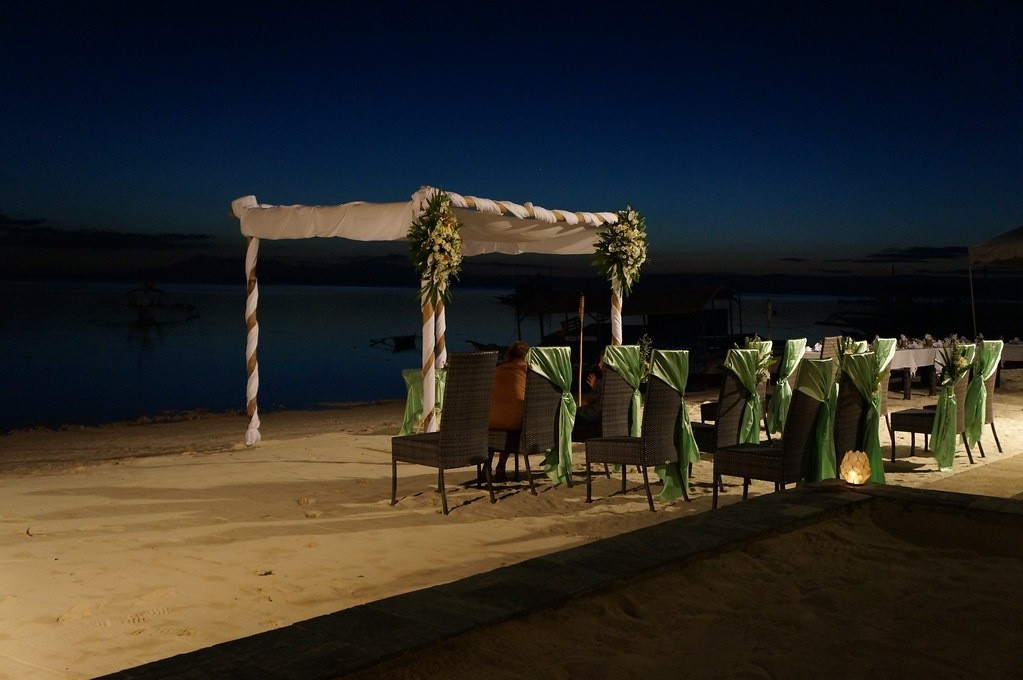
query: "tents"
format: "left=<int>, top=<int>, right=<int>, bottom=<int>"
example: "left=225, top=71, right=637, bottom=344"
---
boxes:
left=969, top=226, right=1023, bottom=342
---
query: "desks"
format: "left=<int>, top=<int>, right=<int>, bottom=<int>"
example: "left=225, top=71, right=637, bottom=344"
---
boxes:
left=397, top=362, right=455, bottom=434
left=991, top=335, right=1023, bottom=392
left=897, top=334, right=976, bottom=396
left=763, top=343, right=829, bottom=398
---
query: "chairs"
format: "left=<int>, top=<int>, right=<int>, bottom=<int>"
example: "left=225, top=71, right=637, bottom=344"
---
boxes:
left=667, top=350, right=760, bottom=492
left=584, top=347, right=689, bottom=516
left=479, top=348, right=577, bottom=498
left=569, top=342, right=642, bottom=478
left=964, top=332, right=1004, bottom=462
left=383, top=348, right=501, bottom=516
left=711, top=328, right=894, bottom=508
left=885, top=342, right=975, bottom=474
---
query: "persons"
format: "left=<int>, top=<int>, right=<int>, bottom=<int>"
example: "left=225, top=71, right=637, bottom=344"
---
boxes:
left=479, top=342, right=531, bottom=482
left=547, top=347, right=607, bottom=457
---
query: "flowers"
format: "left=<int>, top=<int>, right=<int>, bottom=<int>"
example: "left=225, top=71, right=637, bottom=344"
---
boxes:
left=930, top=340, right=970, bottom=391
left=597, top=207, right=649, bottom=297
left=740, top=333, right=776, bottom=385
left=830, top=336, right=862, bottom=381
left=412, top=195, right=466, bottom=309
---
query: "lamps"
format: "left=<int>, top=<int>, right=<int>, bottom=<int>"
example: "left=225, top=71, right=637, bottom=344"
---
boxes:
left=832, top=442, right=874, bottom=500
